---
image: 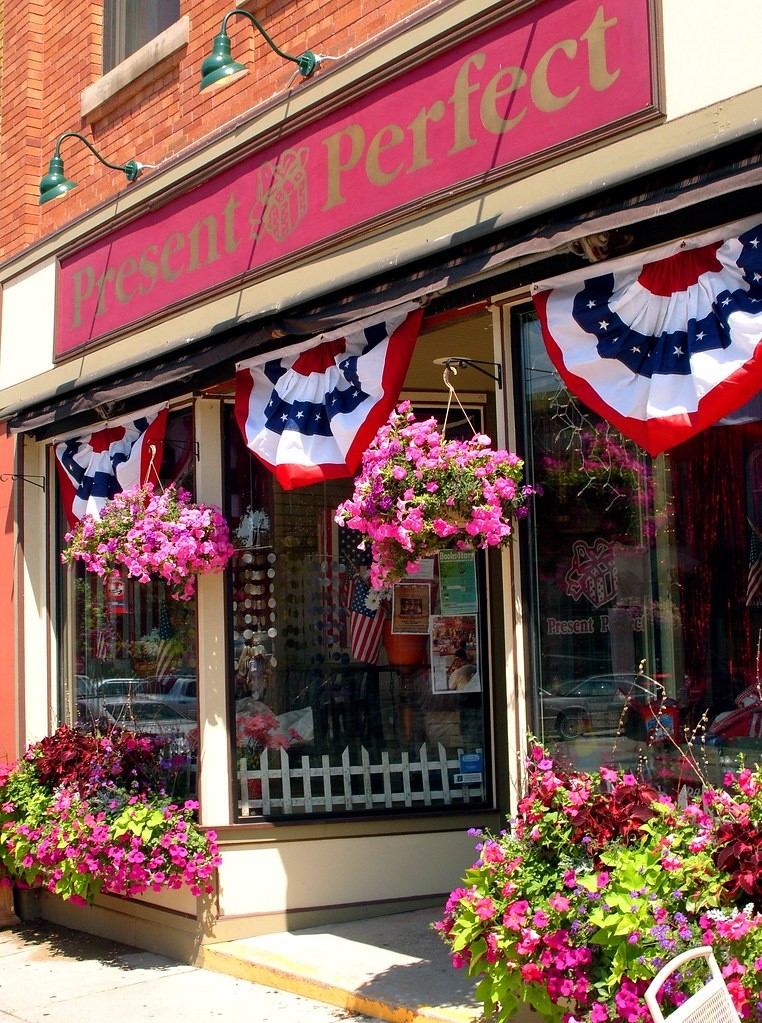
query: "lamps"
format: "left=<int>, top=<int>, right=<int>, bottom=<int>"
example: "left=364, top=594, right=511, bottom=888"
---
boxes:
left=198, top=9, right=320, bottom=94
left=38, top=133, right=142, bottom=205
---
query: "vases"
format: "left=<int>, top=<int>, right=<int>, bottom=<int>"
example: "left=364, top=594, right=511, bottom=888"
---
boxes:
left=545, top=484, right=605, bottom=529
left=382, top=619, right=429, bottom=677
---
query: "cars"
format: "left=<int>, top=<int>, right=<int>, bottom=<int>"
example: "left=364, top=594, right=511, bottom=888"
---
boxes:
left=538, top=671, right=689, bottom=744
left=278, top=666, right=485, bottom=764
left=74, top=672, right=197, bottom=740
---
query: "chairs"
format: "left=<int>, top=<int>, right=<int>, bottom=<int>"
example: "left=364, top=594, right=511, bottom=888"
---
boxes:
left=644, top=946, right=741, bottom=1023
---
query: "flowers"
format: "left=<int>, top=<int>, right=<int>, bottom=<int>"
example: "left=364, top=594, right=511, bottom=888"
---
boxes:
left=335, top=401, right=537, bottom=590
left=0, top=674, right=220, bottom=909
left=378, top=582, right=438, bottom=619
left=57, top=482, right=234, bottom=600
left=545, top=422, right=674, bottom=557
left=431, top=657, right=762, bottom=1023
